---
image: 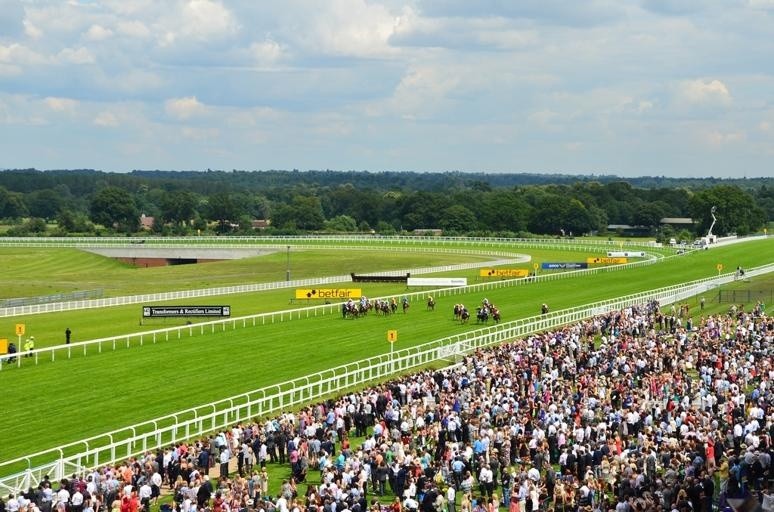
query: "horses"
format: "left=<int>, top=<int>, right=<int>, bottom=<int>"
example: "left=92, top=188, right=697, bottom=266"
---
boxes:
left=341, top=301, right=398, bottom=319
left=428, top=301, right=435, bottom=310
left=403, top=302, right=409, bottom=313
left=454, top=306, right=469, bottom=323
left=542, top=308, right=547, bottom=314
left=475, top=302, right=501, bottom=323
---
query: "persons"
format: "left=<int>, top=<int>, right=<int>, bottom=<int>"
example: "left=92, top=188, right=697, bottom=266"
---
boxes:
left=64, top=327, right=72, bottom=344
left=339, top=293, right=774, bottom=512
left=12, top=344, right=17, bottom=362
left=0, top=396, right=338, bottom=511
left=532, top=272, right=537, bottom=282
left=524, top=272, right=532, bottom=284
left=23, top=339, right=29, bottom=358
left=7, top=343, right=16, bottom=365
left=29, top=336, right=35, bottom=357
left=736, top=265, right=744, bottom=278
